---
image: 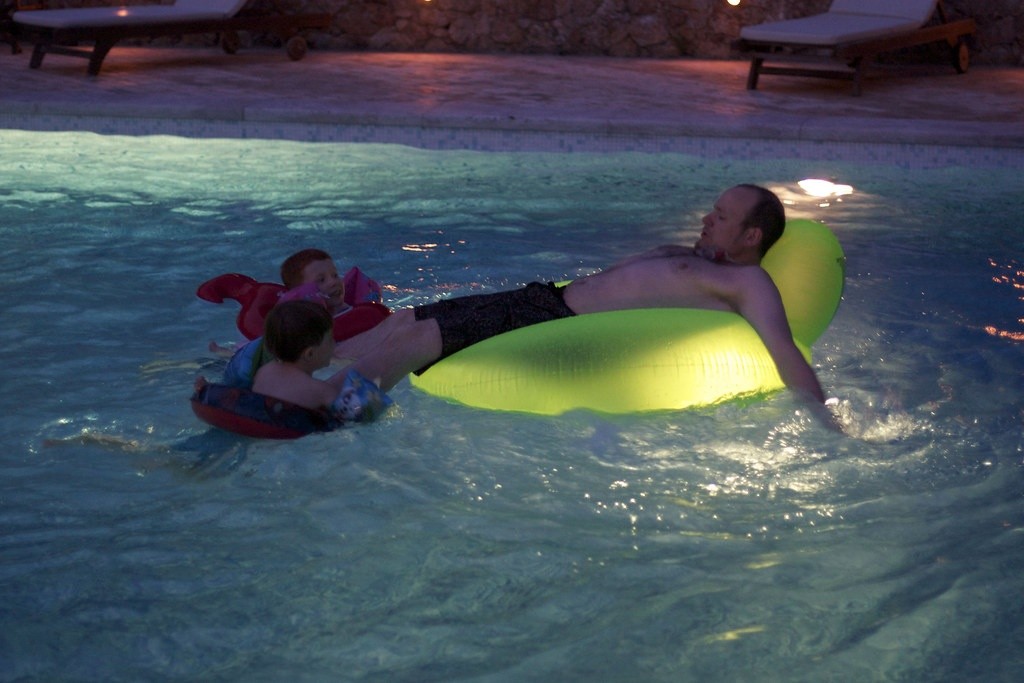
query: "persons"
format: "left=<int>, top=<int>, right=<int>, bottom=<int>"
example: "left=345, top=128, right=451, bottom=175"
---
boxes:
left=280, top=248, right=345, bottom=304
left=250, top=299, right=340, bottom=408
left=336, top=181, right=826, bottom=404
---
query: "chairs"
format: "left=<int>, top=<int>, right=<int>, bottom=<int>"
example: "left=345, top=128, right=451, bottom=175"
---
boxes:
left=737, top=0, right=980, bottom=98
left=9, top=0, right=250, bottom=79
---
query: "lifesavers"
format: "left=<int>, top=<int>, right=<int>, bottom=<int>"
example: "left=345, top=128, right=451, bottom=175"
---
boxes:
left=197, top=266, right=392, bottom=347
left=189, top=335, right=394, bottom=442
